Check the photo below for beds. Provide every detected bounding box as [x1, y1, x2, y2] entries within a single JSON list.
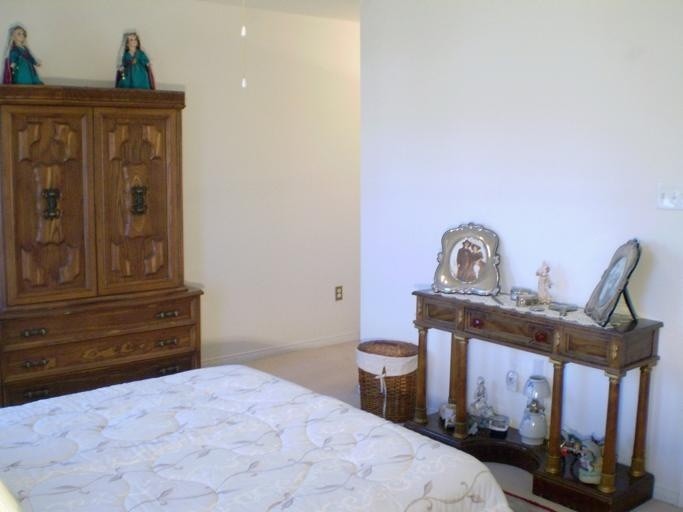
[[0, 364, 513, 511]]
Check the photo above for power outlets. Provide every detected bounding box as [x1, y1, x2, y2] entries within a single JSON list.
[[335, 285, 343, 300]]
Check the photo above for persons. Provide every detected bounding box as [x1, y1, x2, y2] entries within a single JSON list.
[[456, 240, 484, 282], [114, 32, 155, 89], [4, 23, 44, 85], [536, 261, 553, 304]]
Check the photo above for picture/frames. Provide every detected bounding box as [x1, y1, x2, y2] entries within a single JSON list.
[[583, 238, 640, 326], [431, 222, 502, 296]]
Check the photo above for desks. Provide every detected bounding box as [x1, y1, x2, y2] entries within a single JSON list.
[[410, 287, 665, 493]]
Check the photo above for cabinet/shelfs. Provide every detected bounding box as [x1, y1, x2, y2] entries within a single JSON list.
[[1, 82, 201, 412]]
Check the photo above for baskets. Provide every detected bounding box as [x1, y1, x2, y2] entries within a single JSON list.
[[354, 341, 418, 423]]
